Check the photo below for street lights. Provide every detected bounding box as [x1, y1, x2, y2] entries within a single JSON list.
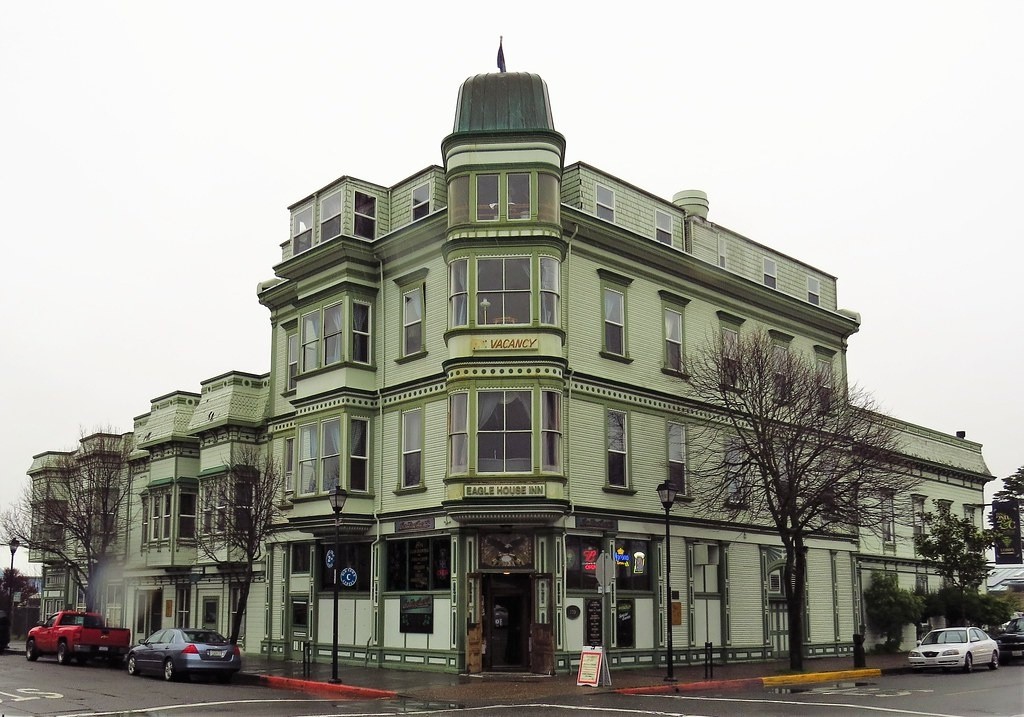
[[327, 485, 348, 685], [656, 479, 681, 683], [7, 538, 21, 620]]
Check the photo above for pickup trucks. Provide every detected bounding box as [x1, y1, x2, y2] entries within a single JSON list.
[[26, 610, 131, 665]]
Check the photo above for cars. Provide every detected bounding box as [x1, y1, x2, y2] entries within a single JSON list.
[[996, 615, 1024, 663], [908, 627, 999, 672], [126, 627, 241, 681]]
